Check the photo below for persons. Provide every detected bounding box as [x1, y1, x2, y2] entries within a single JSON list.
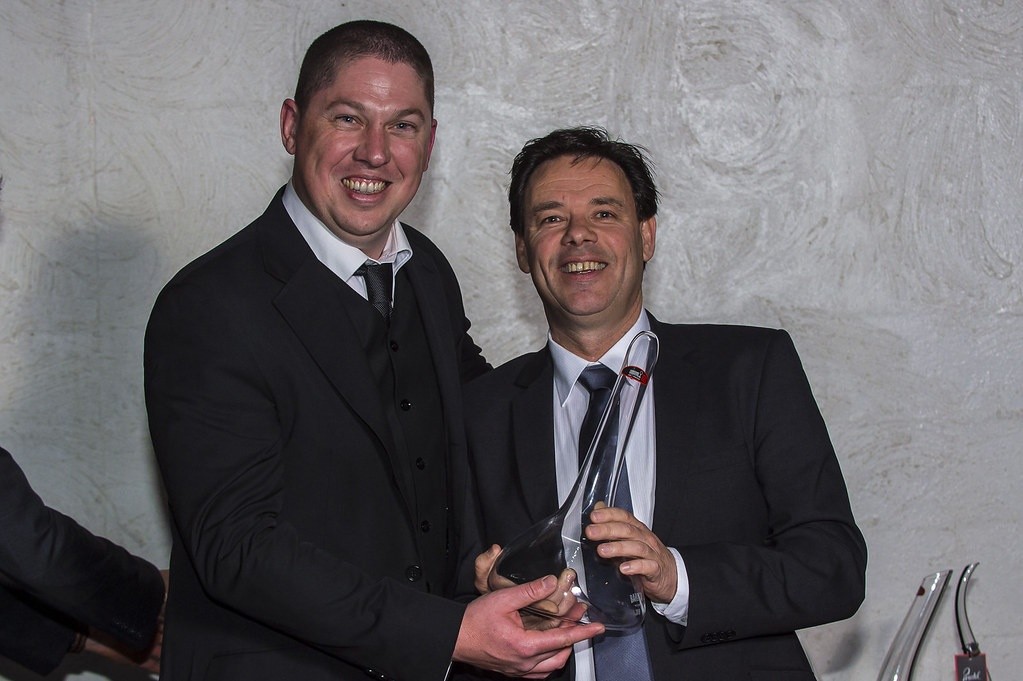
[[0, 447, 165, 676], [462, 126, 867, 681], [144, 20, 606, 681]]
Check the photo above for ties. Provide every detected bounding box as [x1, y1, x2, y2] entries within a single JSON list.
[[576, 364, 653, 681], [352, 263, 393, 324]]
